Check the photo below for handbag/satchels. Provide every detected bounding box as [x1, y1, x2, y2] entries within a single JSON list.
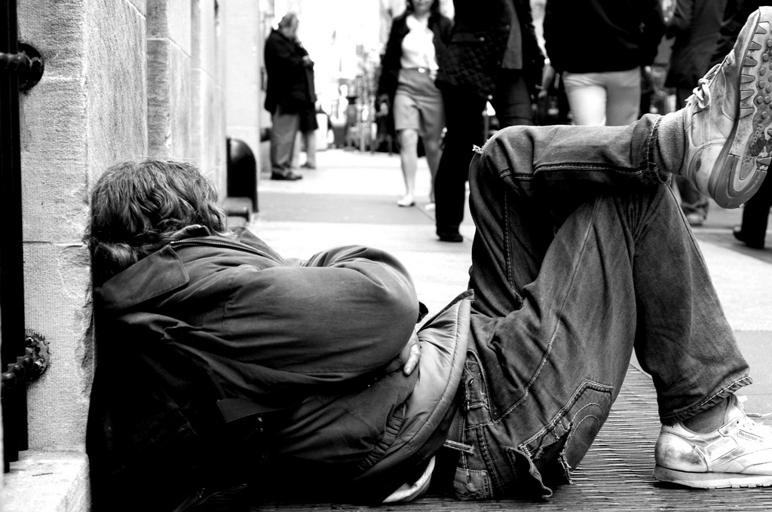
[[89, 317, 277, 511]]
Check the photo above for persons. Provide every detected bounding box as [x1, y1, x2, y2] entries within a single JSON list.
[[541, 0, 666, 127], [92, 4, 772, 507], [263, 12, 319, 183], [373, 1, 453, 208], [732, 158, 771, 251], [434, 0, 544, 243], [661, 0, 772, 227]]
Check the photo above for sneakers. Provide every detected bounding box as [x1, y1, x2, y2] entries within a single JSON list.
[[399, 196, 414, 207], [654, 394, 772, 489], [684, 7, 771, 209], [733, 226, 764, 249], [437, 230, 463, 242], [273, 169, 300, 180]]
